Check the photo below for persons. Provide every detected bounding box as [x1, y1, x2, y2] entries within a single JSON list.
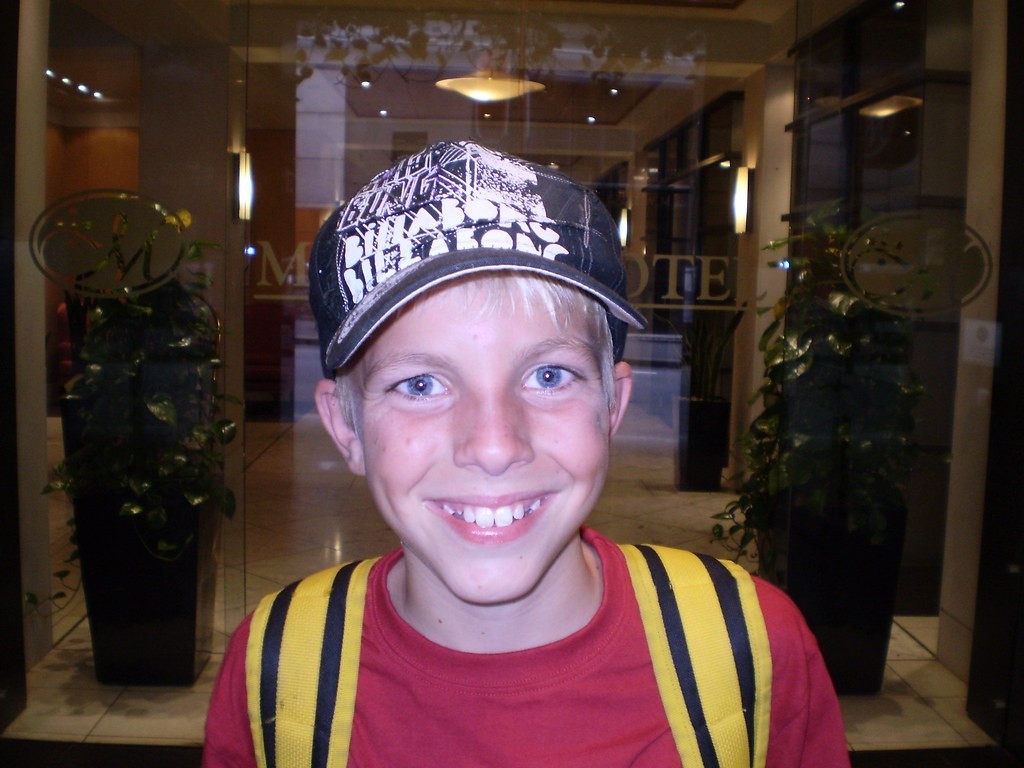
[[199, 142, 850, 768]]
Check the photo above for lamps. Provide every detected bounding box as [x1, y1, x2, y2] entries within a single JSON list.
[[234, 151, 251, 221], [618, 208, 630, 246], [732, 167, 755, 235], [434, 45, 545, 102]]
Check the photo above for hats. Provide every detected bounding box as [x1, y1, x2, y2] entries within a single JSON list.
[[309, 139, 650, 383]]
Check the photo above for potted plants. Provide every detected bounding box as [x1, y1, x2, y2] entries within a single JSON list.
[[24, 229, 240, 685], [708, 196, 928, 695]]
